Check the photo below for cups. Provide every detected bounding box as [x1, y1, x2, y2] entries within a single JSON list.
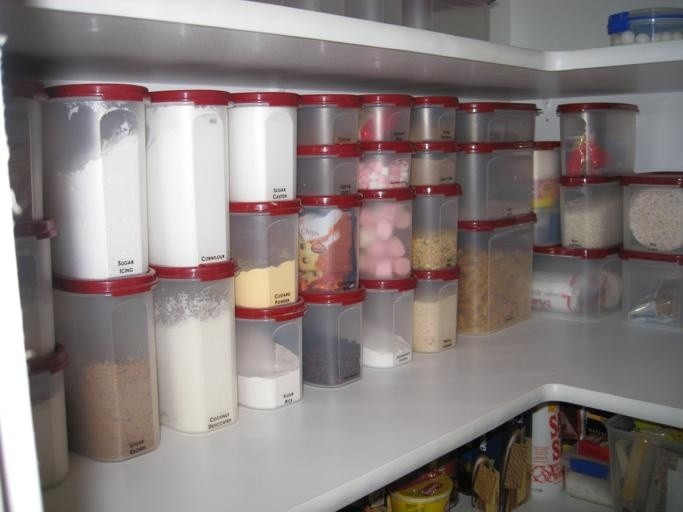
[[387, 471, 455, 512]]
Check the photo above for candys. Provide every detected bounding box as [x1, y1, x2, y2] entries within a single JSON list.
[[357, 158, 411, 190], [359, 203, 413, 277]]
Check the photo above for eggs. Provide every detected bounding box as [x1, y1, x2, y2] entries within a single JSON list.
[[611, 30, 683, 46]]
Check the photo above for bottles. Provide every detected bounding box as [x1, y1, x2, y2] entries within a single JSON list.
[[362, 192, 414, 278], [53, 276, 160, 465], [361, 278, 417, 370], [415, 94, 460, 146], [414, 269, 461, 354], [298, 90, 360, 145], [528, 401, 565, 497], [13, 226, 59, 358], [43, 83, 148, 276], [412, 141, 462, 189], [3, 79, 43, 222], [152, 261, 239, 433], [229, 205, 302, 307], [298, 286, 366, 389], [297, 142, 361, 196], [150, 88, 232, 267], [294, 192, 362, 293], [414, 187, 460, 271], [236, 306, 308, 413], [356, 92, 413, 143], [232, 91, 301, 202], [27, 353, 71, 492], [359, 143, 413, 191]]
